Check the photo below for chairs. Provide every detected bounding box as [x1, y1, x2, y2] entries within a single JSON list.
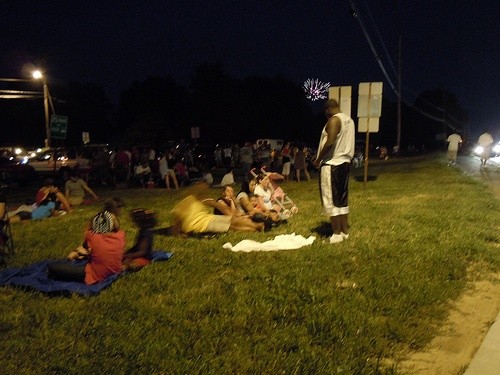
[[0, 206, 15, 265]]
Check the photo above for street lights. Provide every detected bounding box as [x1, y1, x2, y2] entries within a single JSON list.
[[28, 68, 52, 148]]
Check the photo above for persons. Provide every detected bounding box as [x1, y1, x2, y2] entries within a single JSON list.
[[314, 99, 355, 243], [0, 139, 401, 287], [446, 130, 462, 167], [479, 129, 494, 167]]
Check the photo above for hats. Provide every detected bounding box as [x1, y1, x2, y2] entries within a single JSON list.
[[92, 210, 114, 234]]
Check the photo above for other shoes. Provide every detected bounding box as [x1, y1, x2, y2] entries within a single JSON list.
[[340, 231, 350, 239], [322, 234, 343, 245]]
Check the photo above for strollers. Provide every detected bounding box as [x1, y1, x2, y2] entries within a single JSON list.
[[250, 165, 298, 218]]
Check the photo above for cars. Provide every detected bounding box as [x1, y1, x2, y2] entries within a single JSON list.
[[26, 146, 78, 173], [475, 142, 500, 158]]
